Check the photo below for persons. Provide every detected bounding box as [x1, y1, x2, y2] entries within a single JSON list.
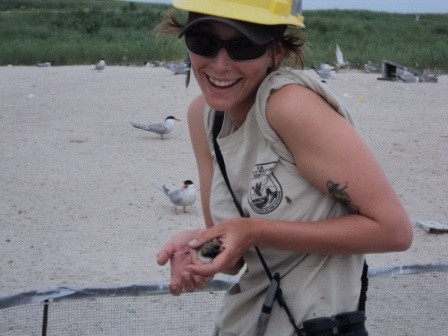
[[148, 2, 415, 336]]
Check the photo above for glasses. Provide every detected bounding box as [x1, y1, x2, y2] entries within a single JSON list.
[[184, 28, 272, 60]]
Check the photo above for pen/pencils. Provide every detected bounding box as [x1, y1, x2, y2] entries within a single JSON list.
[[254, 271, 281, 336]]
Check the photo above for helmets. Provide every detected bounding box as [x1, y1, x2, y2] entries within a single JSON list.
[[170, 0, 306, 27]]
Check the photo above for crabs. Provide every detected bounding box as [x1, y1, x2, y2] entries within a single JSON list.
[[196, 241, 226, 265]]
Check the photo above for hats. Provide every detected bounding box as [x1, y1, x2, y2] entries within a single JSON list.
[[179, 11, 288, 45]]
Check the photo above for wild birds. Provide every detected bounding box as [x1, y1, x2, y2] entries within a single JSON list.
[[130, 115, 184, 140], [90, 58, 106, 71], [312, 60, 349, 84], [163, 180, 197, 215]]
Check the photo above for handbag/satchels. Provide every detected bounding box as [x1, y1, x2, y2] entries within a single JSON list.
[[301, 311, 369, 336]]
